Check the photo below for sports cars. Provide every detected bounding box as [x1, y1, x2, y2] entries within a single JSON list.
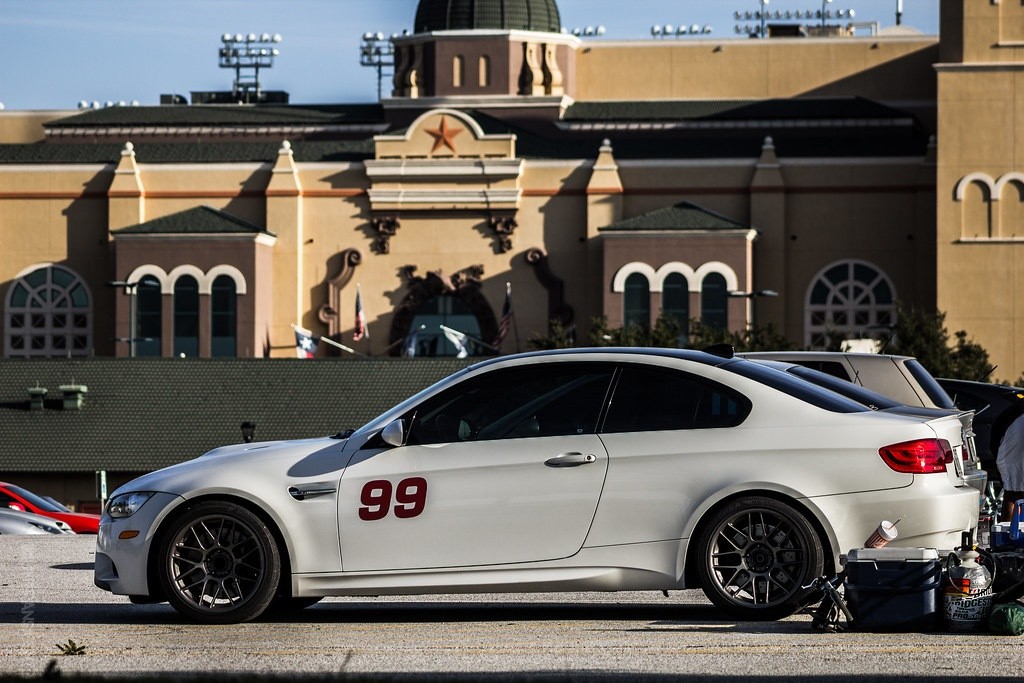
[[92, 344, 978, 624]]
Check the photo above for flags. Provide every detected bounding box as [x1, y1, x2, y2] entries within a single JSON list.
[[493, 295, 512, 350], [294, 330, 320, 358], [352, 293, 365, 343], [444, 328, 468, 358], [404, 333, 417, 358]]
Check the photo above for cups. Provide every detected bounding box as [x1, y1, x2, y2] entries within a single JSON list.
[[865, 520, 897, 548]]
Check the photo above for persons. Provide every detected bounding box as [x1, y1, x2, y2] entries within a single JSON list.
[[996, 403, 1024, 522]]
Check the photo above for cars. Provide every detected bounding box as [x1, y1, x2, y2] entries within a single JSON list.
[[733, 350, 988, 497], [5, 485, 99, 534], [0, 506, 74, 534], [1, 516, 53, 534]]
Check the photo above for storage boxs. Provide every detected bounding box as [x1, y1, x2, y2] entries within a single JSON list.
[[839, 548, 939, 630]]
